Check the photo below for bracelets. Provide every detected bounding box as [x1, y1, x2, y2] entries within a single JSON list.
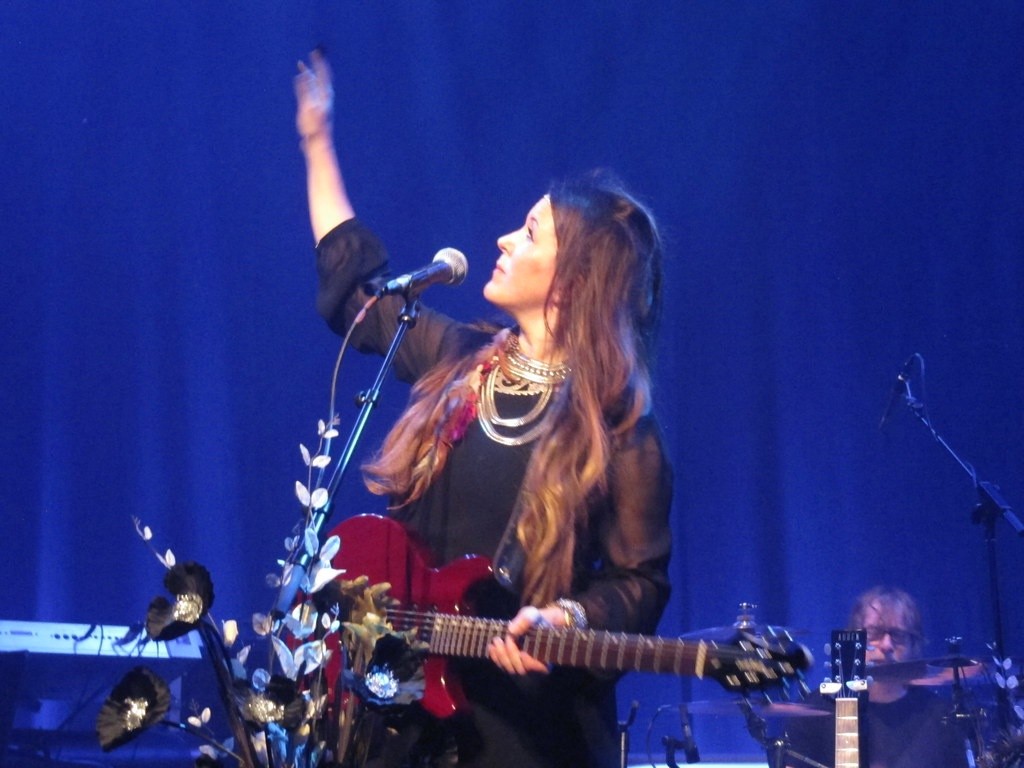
[[549, 598, 588, 632], [297, 132, 321, 148]]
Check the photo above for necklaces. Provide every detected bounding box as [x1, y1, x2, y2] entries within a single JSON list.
[[471, 333, 569, 447]]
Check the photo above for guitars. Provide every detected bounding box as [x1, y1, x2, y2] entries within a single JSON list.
[[820, 627, 874, 768], [284, 514, 813, 720]]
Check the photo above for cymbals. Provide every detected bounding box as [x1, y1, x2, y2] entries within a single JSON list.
[[658, 697, 834, 718], [679, 623, 809, 643], [868, 654, 997, 686]]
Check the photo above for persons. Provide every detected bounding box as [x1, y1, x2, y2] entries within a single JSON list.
[[292, 48, 675, 767], [769, 575, 980, 768]]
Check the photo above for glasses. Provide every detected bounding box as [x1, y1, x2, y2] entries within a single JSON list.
[[865, 625, 916, 645]]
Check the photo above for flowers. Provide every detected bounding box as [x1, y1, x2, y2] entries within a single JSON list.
[[96, 411, 427, 768]]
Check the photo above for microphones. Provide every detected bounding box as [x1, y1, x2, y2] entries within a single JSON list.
[[878, 357, 914, 431], [678, 704, 698, 763], [378, 247, 469, 296]]
